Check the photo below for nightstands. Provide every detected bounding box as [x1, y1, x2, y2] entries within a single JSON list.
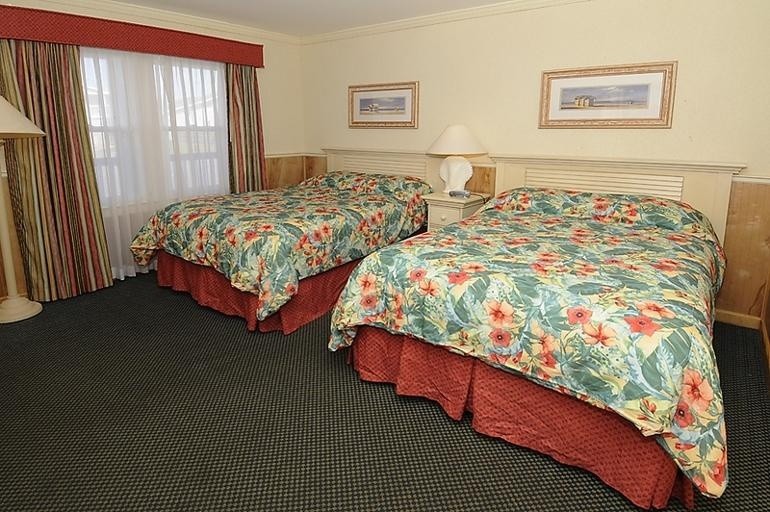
[[420, 190, 491, 232]]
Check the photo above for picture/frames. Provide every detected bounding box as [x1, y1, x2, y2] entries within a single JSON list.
[[348, 81, 419, 130], [536, 62, 679, 129]]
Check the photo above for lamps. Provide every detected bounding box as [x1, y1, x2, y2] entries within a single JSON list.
[[425, 124, 487, 194], [0, 95, 47, 325]]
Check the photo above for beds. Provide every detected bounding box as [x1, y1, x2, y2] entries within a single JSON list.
[[348, 157, 748, 488], [148, 147, 443, 334]]
